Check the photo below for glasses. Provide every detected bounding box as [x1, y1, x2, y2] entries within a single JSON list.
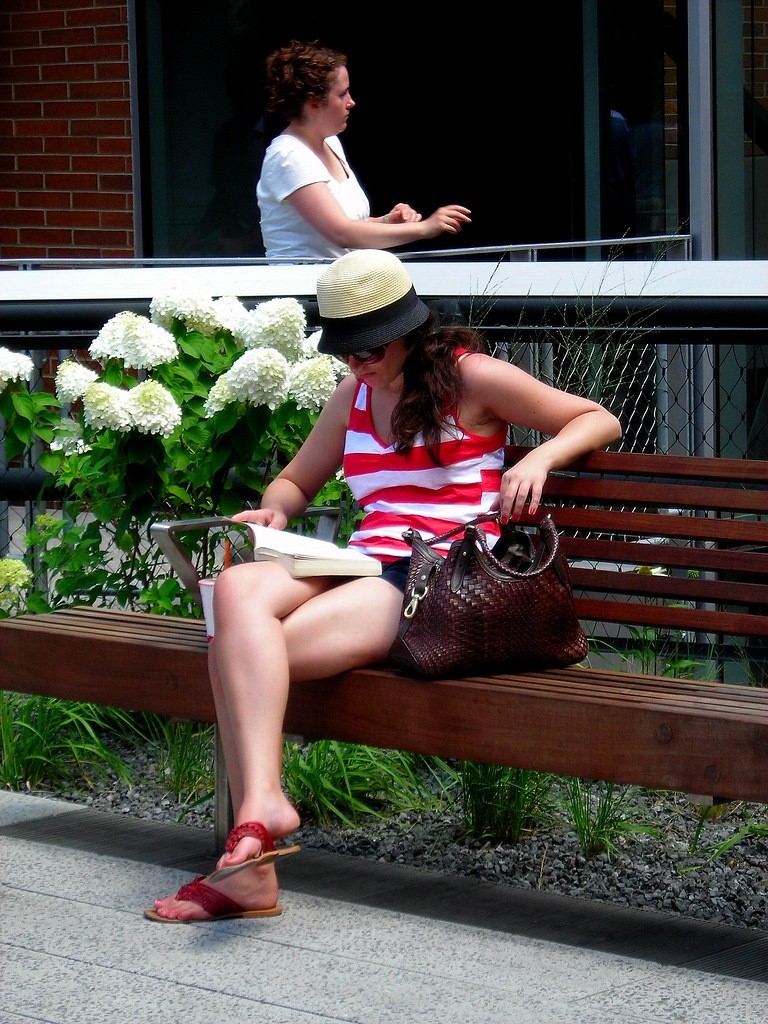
[[332, 342, 397, 364]]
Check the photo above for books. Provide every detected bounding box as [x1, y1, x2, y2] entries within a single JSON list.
[[221, 516, 383, 578]]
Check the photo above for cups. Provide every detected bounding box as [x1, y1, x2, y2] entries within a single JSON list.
[[197, 578, 214, 644]]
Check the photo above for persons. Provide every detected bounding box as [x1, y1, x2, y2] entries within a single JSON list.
[[250, 39, 474, 263], [142, 250, 624, 923]]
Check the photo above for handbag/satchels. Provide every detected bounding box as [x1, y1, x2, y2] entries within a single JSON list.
[[384, 510, 592, 683]]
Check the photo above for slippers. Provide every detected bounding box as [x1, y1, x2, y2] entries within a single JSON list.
[[208, 821, 302, 885], [144, 875, 282, 924]]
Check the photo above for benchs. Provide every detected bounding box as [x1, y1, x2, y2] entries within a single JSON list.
[[0, 442, 768, 858]]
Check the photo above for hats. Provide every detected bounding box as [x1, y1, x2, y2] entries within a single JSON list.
[[318, 247, 430, 352]]
